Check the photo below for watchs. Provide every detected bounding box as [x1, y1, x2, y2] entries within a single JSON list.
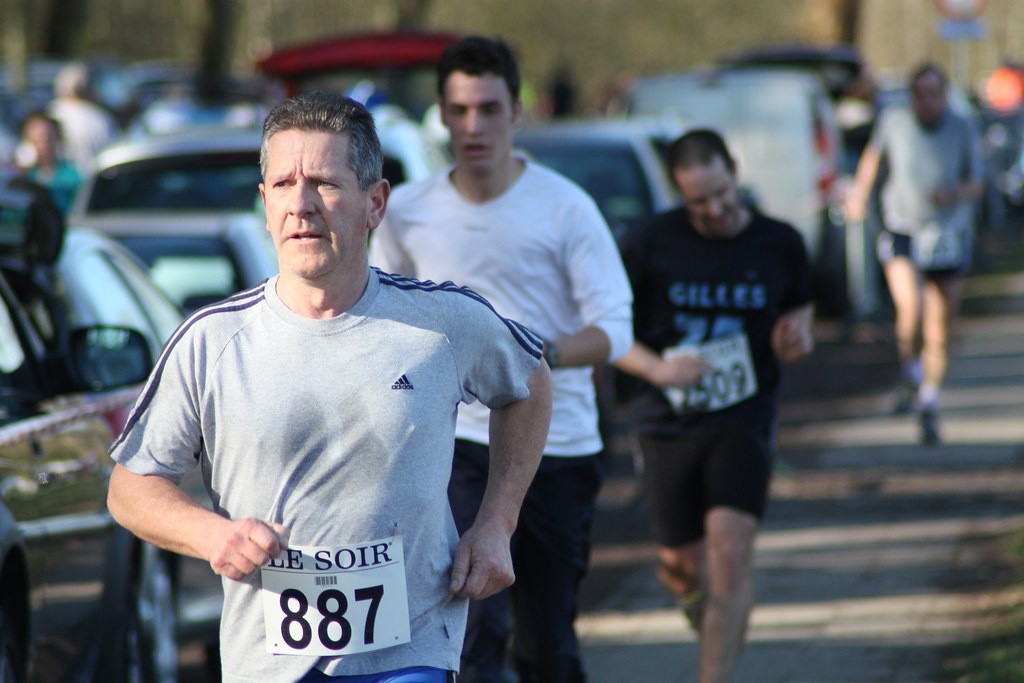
[[544, 341, 560, 369]]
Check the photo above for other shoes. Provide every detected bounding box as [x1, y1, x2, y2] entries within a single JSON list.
[[915, 398, 940, 446], [894, 360, 921, 414], [683, 582, 707, 635]]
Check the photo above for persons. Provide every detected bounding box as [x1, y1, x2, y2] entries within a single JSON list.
[[612, 130, 816, 683], [106, 90, 553, 683], [834, 64, 878, 152], [22, 111, 81, 216], [850, 67, 985, 445], [46, 62, 118, 166], [370, 35, 634, 683]]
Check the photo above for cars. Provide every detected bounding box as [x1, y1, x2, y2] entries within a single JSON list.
[[1, 29, 1024, 683]]
